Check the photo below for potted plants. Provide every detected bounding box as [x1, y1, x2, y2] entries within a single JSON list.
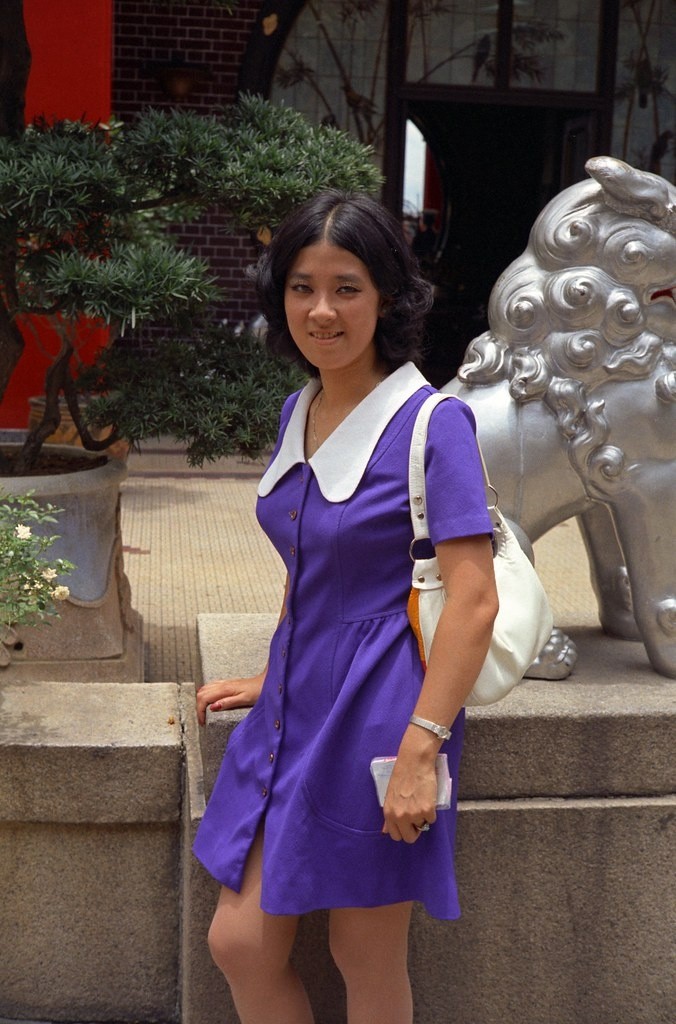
[[1, 91, 390, 603]]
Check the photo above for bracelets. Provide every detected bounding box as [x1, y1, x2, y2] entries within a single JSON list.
[[408, 715, 451, 740]]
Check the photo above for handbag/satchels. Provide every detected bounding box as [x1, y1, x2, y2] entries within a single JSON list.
[[407, 392, 553, 709]]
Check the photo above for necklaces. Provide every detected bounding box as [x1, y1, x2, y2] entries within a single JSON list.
[[311, 374, 386, 451]]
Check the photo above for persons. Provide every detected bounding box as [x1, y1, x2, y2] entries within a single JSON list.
[[195, 186, 500, 1023]]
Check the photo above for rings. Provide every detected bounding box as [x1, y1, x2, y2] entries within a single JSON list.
[[417, 823, 430, 832]]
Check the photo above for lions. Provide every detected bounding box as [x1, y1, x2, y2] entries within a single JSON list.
[[440, 157, 676, 680]]
